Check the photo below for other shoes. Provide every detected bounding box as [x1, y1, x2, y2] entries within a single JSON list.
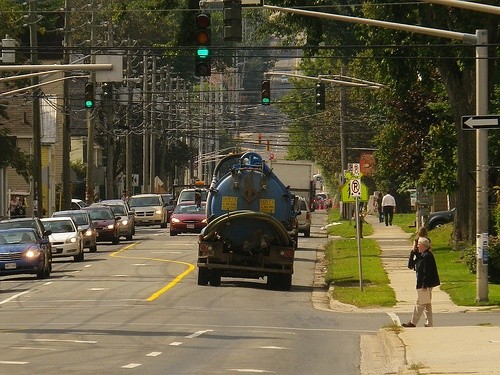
[[402, 321, 416, 327], [424, 323, 428, 327]]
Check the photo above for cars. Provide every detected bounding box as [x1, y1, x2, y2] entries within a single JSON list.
[[427, 207, 455, 231], [296, 198, 312, 238], [70, 188, 208, 245], [168, 201, 208, 237], [51, 210, 98, 252], [0, 214, 53, 279], [38, 217, 87, 262]]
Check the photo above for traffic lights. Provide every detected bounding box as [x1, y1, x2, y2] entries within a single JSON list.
[[102, 82, 114, 101], [83, 81, 95, 111], [194, 12, 212, 77], [261, 79, 271, 105]]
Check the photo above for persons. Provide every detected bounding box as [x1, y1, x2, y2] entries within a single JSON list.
[[382, 190, 395, 226], [8, 194, 19, 219], [413, 227, 432, 253], [359, 202, 364, 213], [377, 192, 384, 223], [401, 237, 440, 327], [19, 195, 28, 218]]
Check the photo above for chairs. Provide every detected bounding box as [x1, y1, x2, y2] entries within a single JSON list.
[[63, 225, 73, 230], [181, 208, 187, 213], [0, 235, 7, 245], [77, 218, 83, 223], [22, 233, 31, 241], [115, 208, 120, 213], [102, 211, 109, 217]]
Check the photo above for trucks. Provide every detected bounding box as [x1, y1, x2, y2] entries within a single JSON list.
[[196, 151, 299, 292], [269, 159, 312, 208]]
